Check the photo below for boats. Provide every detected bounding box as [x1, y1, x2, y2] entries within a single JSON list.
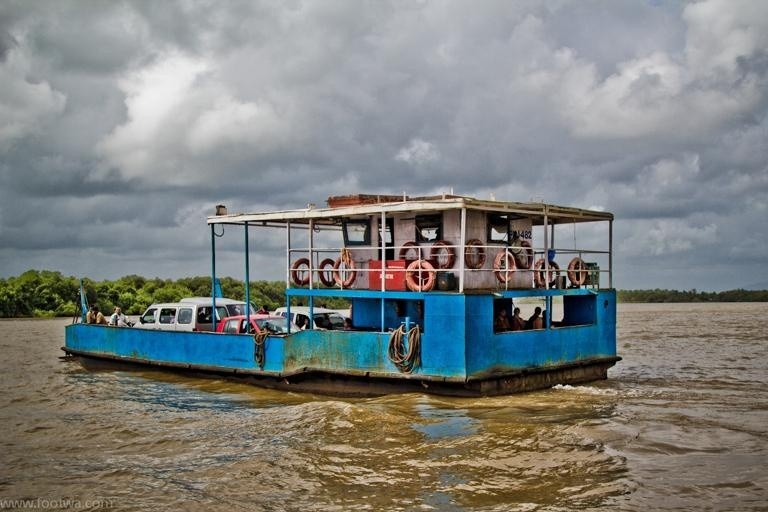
[[60, 194, 621, 400]]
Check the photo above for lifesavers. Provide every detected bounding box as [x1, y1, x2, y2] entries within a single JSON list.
[[429, 240, 455, 269], [405, 260, 437, 292], [333, 257, 356, 287], [399, 242, 423, 269], [319, 258, 338, 287], [534, 259, 560, 286], [291, 258, 314, 285], [515, 241, 533, 269], [465, 239, 486, 271], [567, 258, 586, 286], [494, 251, 517, 283]]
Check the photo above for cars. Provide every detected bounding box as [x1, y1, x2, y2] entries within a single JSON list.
[[131, 296, 348, 337]]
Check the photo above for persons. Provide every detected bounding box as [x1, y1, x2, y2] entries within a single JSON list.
[[91, 306, 109, 326], [296, 302, 304, 306], [110, 306, 131, 327], [85, 304, 95, 325], [319, 303, 327, 308], [237, 311, 351, 337], [511, 307, 524, 330], [363, 222, 383, 242], [415, 225, 427, 241], [143, 306, 217, 324], [541, 310, 554, 330], [526, 306, 543, 330], [493, 308, 512, 332], [254, 305, 269, 314]]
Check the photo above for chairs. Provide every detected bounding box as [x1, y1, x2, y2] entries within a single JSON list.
[[297, 317, 333, 330], [226, 323, 283, 333], [161, 312, 217, 323]]
